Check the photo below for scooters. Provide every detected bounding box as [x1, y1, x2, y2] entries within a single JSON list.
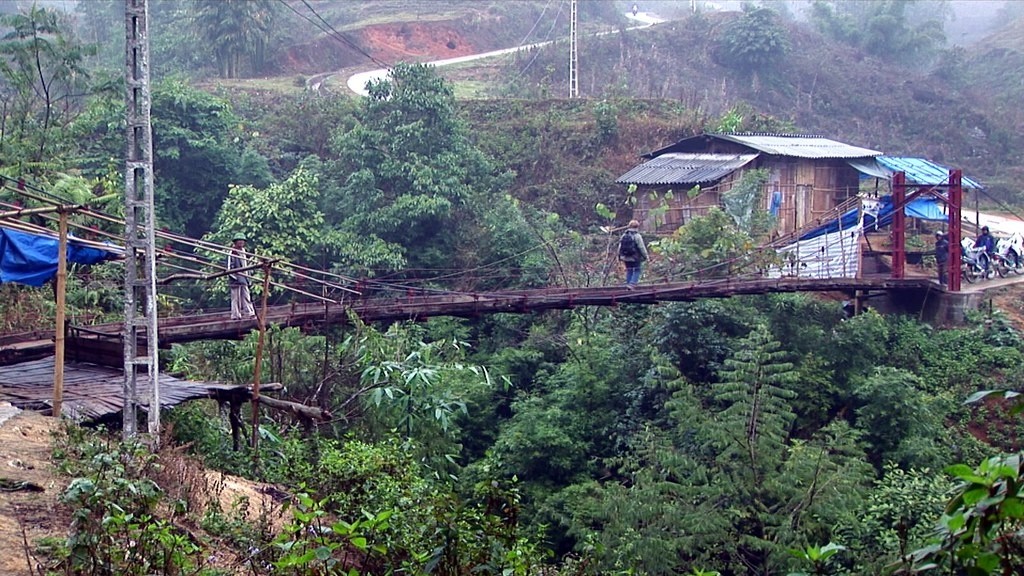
[[961, 239, 1024, 285]]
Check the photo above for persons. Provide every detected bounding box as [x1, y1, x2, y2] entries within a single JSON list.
[[973, 225, 995, 279], [616, 220, 650, 292], [226, 232, 258, 321], [935, 230, 949, 284]]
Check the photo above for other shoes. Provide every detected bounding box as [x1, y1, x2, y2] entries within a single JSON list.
[[626, 285, 635, 291], [250, 315, 257, 319]]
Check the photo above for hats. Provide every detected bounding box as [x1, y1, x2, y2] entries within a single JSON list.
[[232, 233, 248, 242]]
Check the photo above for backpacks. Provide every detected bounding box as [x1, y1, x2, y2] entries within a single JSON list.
[[620, 230, 637, 257]]
